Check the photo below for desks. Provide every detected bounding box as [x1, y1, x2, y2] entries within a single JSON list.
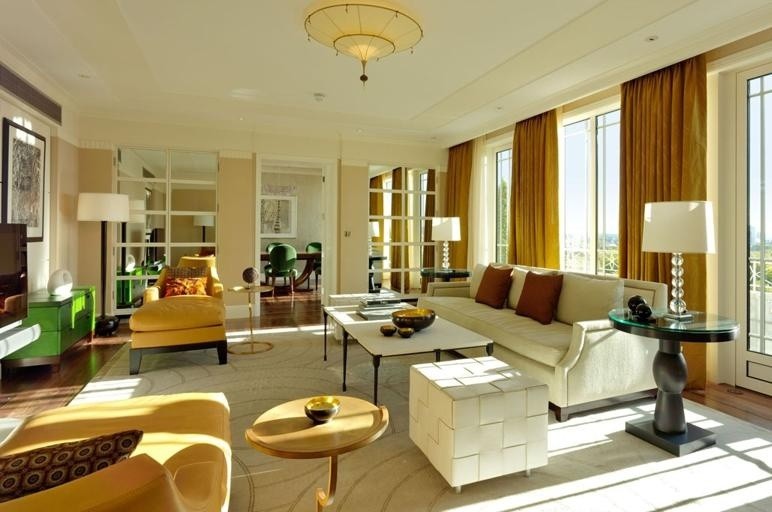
[[421, 268, 472, 282], [607, 309, 739, 455], [244, 395, 389, 511], [228, 285, 275, 354], [178, 257, 217, 268], [260, 253, 387, 292], [323, 302, 494, 406]]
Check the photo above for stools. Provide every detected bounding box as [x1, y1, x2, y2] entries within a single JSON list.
[[409, 357, 549, 494]]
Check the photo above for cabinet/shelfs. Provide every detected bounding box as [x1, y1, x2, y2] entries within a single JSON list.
[[0, 286, 96, 373]]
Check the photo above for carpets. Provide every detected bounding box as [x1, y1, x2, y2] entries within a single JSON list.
[[65, 323, 771, 512]]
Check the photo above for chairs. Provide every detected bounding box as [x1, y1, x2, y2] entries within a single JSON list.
[[264, 244, 297, 294], [306, 243, 321, 288], [314, 268, 321, 290], [0, 391, 230, 509], [263, 242, 287, 286], [129, 266, 228, 373]]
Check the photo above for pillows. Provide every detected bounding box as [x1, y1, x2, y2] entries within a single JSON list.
[[165, 267, 208, 278], [475, 264, 513, 309], [0, 431, 143, 503], [515, 271, 564, 325], [163, 277, 208, 296], [470, 264, 514, 299], [555, 273, 624, 324], [508, 266, 558, 310]]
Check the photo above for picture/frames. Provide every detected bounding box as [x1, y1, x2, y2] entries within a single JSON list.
[[260, 195, 298, 238], [2, 117, 45, 242]]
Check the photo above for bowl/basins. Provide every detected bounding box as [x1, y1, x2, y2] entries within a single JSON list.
[[380, 325, 397, 337], [398, 327, 415, 338], [391, 308, 436, 332], [303, 396, 341, 424]]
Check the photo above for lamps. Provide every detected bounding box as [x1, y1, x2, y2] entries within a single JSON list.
[[369, 221, 380, 256], [192, 216, 214, 242], [431, 216, 462, 272], [303, 1, 425, 86], [641, 200, 717, 320], [77, 192, 129, 336]]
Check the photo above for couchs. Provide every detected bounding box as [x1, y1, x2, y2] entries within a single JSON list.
[[418, 263, 670, 421]]
